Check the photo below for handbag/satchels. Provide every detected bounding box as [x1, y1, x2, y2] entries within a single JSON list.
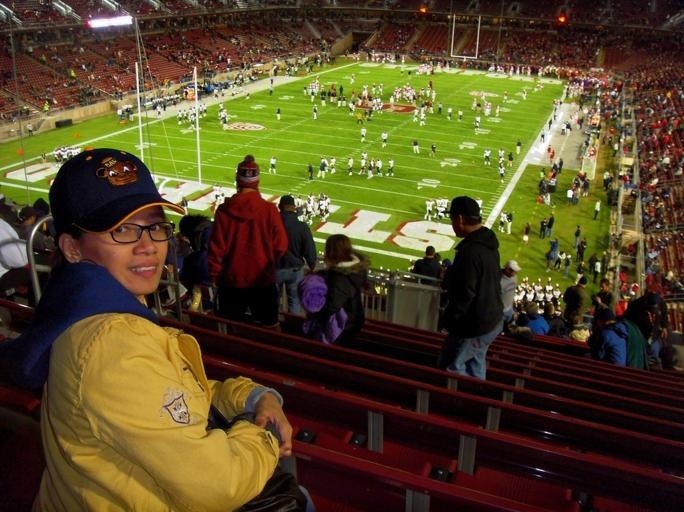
[[238, 466, 307, 512]]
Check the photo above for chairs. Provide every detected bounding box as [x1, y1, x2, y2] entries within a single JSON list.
[[2, 3, 337, 125], [2, 198, 681, 511], [632, 83, 683, 346], [333, 1, 683, 92]]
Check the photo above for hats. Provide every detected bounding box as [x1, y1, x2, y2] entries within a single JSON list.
[[441, 196, 480, 216], [279, 195, 294, 209], [505, 260, 523, 272], [236, 155, 259, 189], [49, 149, 185, 234]]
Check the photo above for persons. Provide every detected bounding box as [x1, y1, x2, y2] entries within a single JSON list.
[[143, 154, 505, 380], [0, 1, 684, 181], [1, 145, 293, 512], [497, 178, 683, 369], [1, 195, 57, 325]]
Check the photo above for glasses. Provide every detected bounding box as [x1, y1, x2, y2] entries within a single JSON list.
[[110, 222, 174, 243]]
